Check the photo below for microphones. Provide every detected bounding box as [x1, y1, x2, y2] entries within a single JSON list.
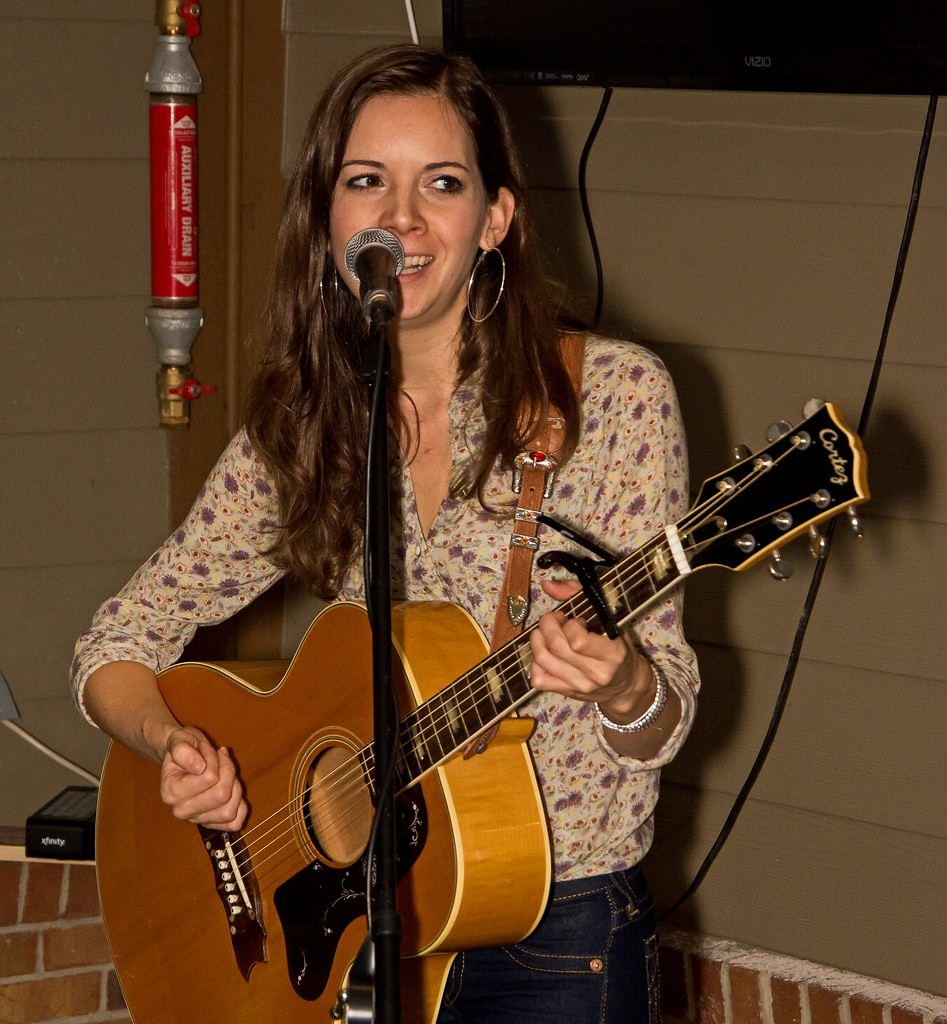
[[344, 228, 405, 320]]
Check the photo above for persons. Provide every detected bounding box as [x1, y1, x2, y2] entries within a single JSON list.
[[68, 41, 702, 1024]]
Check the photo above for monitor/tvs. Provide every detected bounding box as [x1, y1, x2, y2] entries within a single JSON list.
[[443, 0, 947, 96]]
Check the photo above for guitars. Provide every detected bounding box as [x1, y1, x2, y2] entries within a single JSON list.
[[94, 399, 870, 1024]]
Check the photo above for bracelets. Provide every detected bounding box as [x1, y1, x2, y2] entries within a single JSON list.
[[594, 662, 669, 734]]
